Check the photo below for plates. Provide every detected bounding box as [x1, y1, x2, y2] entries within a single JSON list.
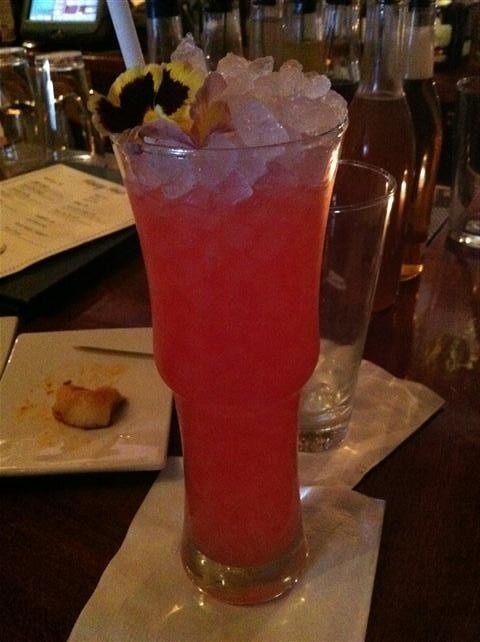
[[0, 323, 177, 472]]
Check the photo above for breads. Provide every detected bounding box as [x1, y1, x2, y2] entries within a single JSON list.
[[52, 379, 128, 428]]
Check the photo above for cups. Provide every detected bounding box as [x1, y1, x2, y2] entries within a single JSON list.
[[35, 48, 105, 162], [0, 45, 48, 174], [445, 76, 480, 250], [113, 102, 350, 607], [301, 148, 398, 458]]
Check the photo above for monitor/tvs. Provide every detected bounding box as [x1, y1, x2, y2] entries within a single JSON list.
[[21, 0, 104, 30]]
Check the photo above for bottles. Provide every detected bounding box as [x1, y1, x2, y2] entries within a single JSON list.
[[147, 0, 443, 311]]
